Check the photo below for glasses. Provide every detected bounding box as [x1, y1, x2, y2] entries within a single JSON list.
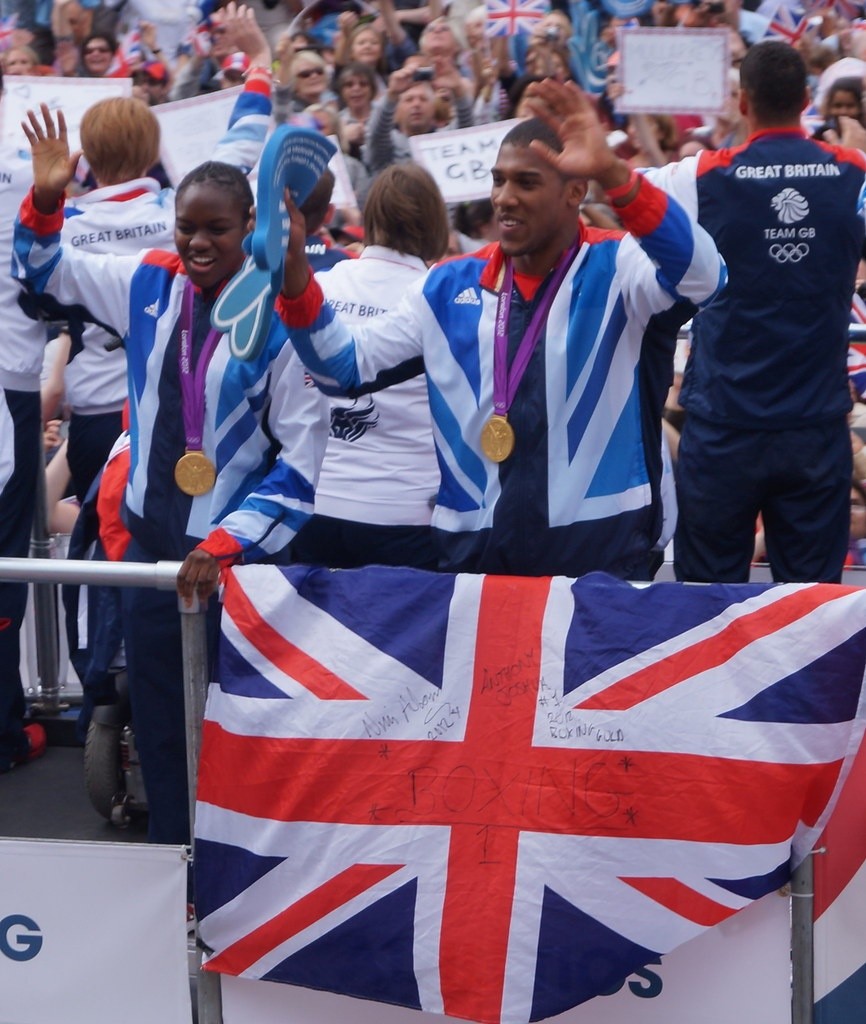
[[296, 67, 325, 78], [850, 504, 866, 515], [84, 46, 112, 53]]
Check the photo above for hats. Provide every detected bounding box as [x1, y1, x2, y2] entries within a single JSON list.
[[221, 52, 250, 73], [131, 61, 169, 82]]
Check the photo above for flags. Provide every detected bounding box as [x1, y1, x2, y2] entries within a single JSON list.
[[197, 558, 866, 1024]]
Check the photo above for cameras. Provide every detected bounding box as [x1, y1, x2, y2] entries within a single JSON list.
[[414, 67, 435, 82]]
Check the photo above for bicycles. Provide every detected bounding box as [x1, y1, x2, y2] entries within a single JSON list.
[[82, 663, 154, 834]]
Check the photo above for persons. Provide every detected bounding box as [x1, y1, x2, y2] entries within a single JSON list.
[[2, 0, 866, 811]]
